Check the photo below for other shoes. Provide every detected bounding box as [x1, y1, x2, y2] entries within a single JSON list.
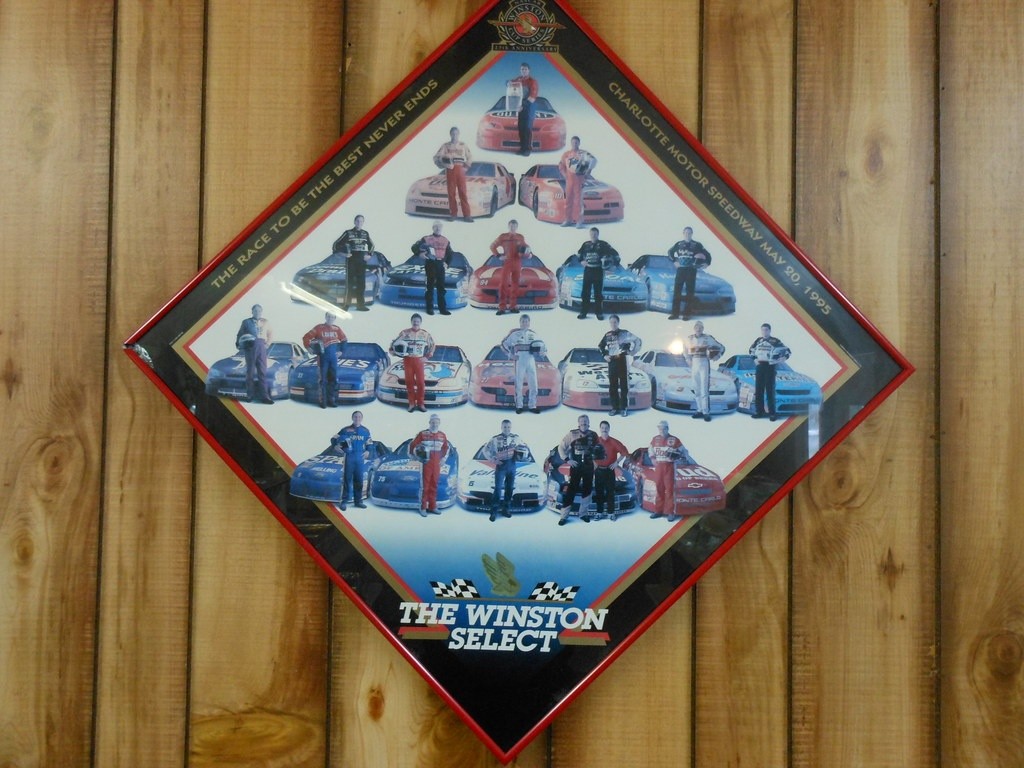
[[340, 501, 676, 527], [517, 149, 531, 157], [342, 303, 691, 321], [246, 394, 777, 425], [560, 220, 583, 228], [447, 215, 475, 223]]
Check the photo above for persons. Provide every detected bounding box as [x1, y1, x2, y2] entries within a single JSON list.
[[500, 314, 547, 414], [433, 127, 474, 222], [749, 324, 792, 422], [682, 321, 725, 421], [331, 411, 373, 511], [389, 313, 435, 413], [483, 419, 532, 521], [411, 221, 454, 315], [490, 220, 533, 315], [558, 414, 607, 525], [408, 415, 448, 516], [235, 304, 275, 405], [594, 421, 628, 521], [667, 227, 712, 321], [649, 421, 683, 522], [302, 310, 347, 408], [598, 315, 642, 417], [506, 63, 538, 156], [577, 227, 621, 320], [559, 136, 597, 229], [333, 215, 375, 311]]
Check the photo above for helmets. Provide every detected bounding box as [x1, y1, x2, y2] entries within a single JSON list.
[[592, 443, 607, 460], [310, 339, 325, 355], [439, 154, 455, 170], [529, 340, 545, 356], [337, 242, 353, 258], [692, 252, 707, 270], [419, 243, 437, 261], [337, 436, 353, 454], [600, 254, 615, 270], [769, 346, 788, 365], [392, 340, 410, 358], [705, 345, 721, 360], [515, 445, 530, 463], [414, 445, 430, 464], [238, 333, 258, 350], [618, 341, 635, 355], [519, 244, 531, 259]]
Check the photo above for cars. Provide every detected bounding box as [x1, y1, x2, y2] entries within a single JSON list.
[[288, 343, 391, 403], [379, 252, 473, 310], [469, 251, 558, 309], [289, 441, 392, 502], [543, 445, 636, 516], [634, 348, 738, 415], [376, 345, 472, 407], [477, 96, 566, 152], [457, 442, 548, 513], [518, 163, 624, 224], [623, 447, 726, 515], [555, 253, 648, 311], [626, 254, 736, 312], [404, 161, 516, 218], [558, 348, 652, 410], [370, 438, 459, 508], [469, 344, 563, 407], [717, 354, 823, 416], [205, 342, 309, 399], [289, 250, 392, 306]]
